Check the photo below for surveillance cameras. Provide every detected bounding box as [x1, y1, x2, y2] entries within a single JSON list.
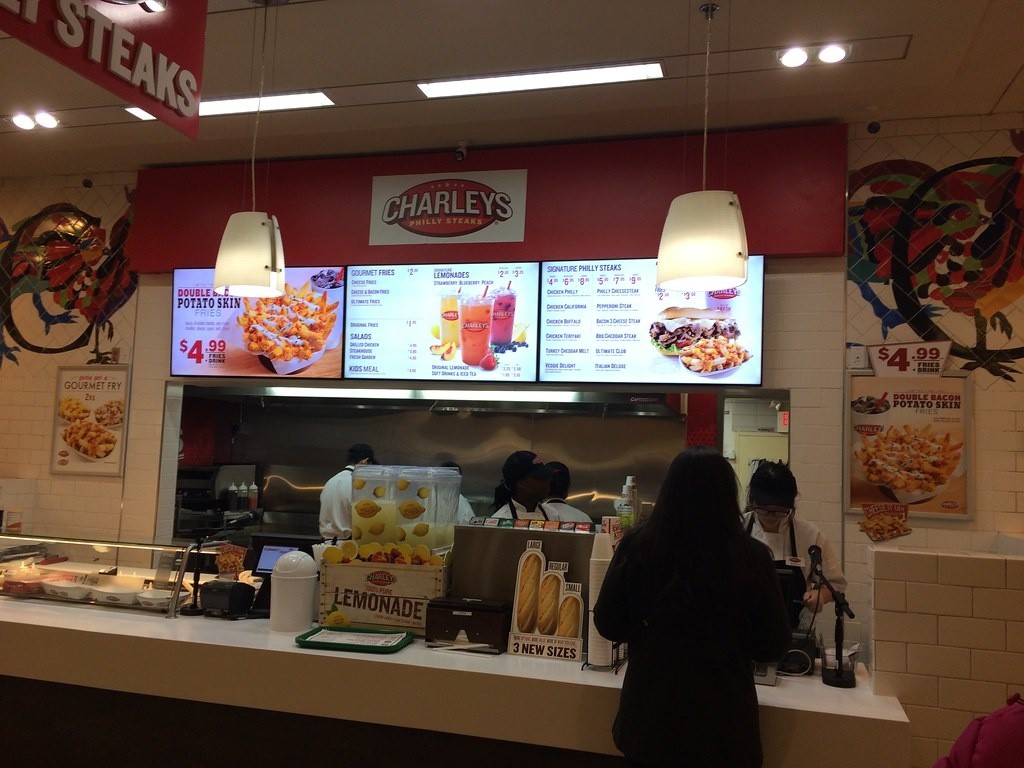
[[453, 147, 466, 160]]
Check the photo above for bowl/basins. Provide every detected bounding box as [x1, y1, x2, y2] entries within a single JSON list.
[[680, 346, 753, 379], [136, 589, 191, 613], [256, 347, 324, 375], [90, 586, 141, 605], [56, 412, 124, 462], [41, 580, 91, 600]]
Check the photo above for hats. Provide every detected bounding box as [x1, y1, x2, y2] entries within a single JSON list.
[[502, 449, 552, 481], [351, 443, 381, 466]]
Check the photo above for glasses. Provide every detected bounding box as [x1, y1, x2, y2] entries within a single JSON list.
[[753, 503, 791, 518]]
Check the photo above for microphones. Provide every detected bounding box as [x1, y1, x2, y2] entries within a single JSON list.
[[808, 544, 823, 574], [228, 511, 259, 526]]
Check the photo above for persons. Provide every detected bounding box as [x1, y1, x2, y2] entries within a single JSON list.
[[489, 451, 561, 521], [592, 447, 793, 767], [739, 461, 848, 635], [319, 444, 374, 543], [440, 462, 476, 523], [545, 461, 594, 523], [932, 693, 1024, 768]]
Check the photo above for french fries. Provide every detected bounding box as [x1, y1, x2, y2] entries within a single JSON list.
[[681, 336, 750, 372], [238, 282, 339, 360], [855, 424, 963, 492], [58, 396, 116, 457]]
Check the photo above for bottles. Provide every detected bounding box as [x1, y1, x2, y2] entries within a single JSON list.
[[616, 476, 642, 529], [227, 482, 238, 510], [238, 482, 248, 511], [249, 482, 258, 510]]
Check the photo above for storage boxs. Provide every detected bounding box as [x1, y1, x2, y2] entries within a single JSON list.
[[319, 562, 445, 637]]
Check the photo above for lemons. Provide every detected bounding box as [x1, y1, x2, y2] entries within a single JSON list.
[[325, 611, 351, 626], [322, 478, 444, 565]]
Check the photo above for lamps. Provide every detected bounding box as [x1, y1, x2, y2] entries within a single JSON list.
[[657, 4, 749, 293], [214, 0, 287, 299]]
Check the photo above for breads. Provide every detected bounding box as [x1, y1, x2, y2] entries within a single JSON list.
[[517, 554, 580, 638]]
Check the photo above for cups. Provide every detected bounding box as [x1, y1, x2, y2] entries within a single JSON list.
[[588, 516, 628, 666], [440, 290, 517, 366], [310, 274, 345, 349], [851, 400, 891, 446]]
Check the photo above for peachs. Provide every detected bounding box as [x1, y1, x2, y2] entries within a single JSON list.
[[429, 342, 456, 360]]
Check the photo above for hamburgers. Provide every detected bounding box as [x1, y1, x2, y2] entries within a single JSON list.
[[649, 306, 741, 355]]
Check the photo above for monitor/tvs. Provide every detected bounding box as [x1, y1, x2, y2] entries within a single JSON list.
[[251, 545, 301, 577]]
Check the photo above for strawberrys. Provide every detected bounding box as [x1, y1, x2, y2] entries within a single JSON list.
[[480, 355, 497, 370]]
